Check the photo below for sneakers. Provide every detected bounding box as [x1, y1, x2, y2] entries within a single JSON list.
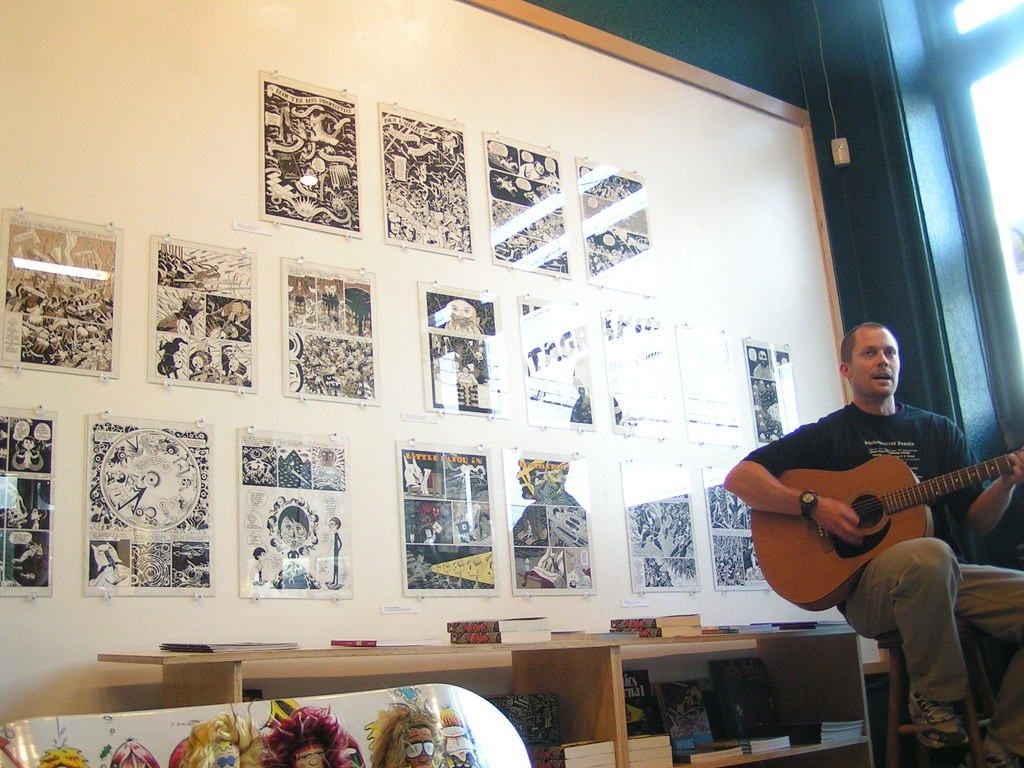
[[973, 736, 1021, 768], [907, 688, 969, 749]]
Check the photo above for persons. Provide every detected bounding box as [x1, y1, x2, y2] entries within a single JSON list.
[[722, 319, 1023, 767], [512, 458, 588, 554]]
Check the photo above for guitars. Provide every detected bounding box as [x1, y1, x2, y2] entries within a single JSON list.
[[751, 448, 1024, 618]]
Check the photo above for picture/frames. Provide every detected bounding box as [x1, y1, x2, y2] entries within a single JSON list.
[[0, 69, 798, 599]]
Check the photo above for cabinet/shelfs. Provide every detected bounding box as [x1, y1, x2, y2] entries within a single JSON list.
[[97, 622, 877, 768]]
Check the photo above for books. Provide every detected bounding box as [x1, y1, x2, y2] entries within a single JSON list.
[[447, 613, 702, 645], [701, 620, 856, 636], [158, 642, 302, 654], [242, 688, 263, 702], [488, 693, 618, 768], [330, 639, 429, 647], [622, 655, 864, 768]]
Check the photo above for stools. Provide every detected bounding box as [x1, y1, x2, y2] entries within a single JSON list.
[[876, 617, 998, 768]]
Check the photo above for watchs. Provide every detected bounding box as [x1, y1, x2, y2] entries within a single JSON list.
[[799, 490, 819, 521]]
